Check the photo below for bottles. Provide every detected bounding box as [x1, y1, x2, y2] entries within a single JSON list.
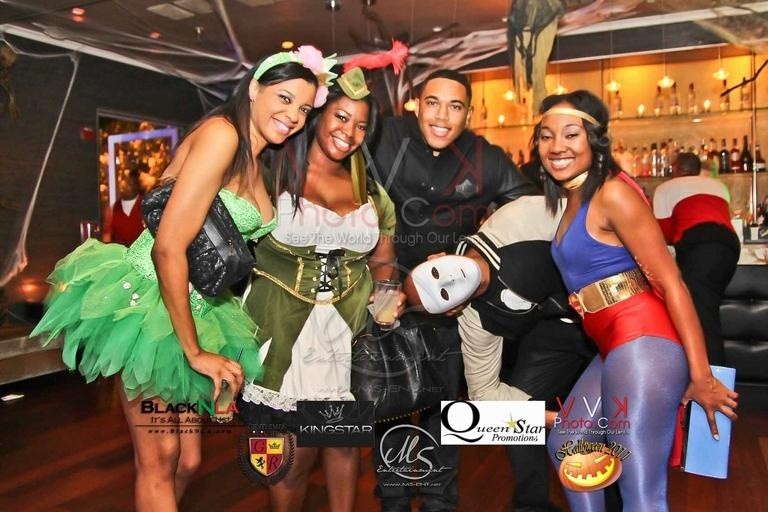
[[654, 86, 665, 116], [505, 134, 767, 175], [741, 78, 750, 108], [720, 79, 731, 111], [80, 215, 97, 242], [687, 83, 697, 113], [614, 90, 622, 120], [479, 96, 489, 129], [670, 82, 681, 114], [520, 96, 530, 130]]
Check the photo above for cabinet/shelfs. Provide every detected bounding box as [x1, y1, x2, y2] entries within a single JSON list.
[[475, 107, 767, 184]]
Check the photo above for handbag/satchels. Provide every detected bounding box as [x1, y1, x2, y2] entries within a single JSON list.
[[141, 181, 253, 297], [348, 313, 463, 427]]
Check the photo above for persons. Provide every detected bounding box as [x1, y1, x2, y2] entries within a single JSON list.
[[527, 90, 739, 512], [339, 70, 545, 512], [32, 50, 319, 512], [652, 151, 741, 368], [235, 70, 407, 511], [404, 195, 627, 512]]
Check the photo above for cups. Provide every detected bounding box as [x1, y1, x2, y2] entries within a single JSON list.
[[374, 280, 400, 326], [216, 378, 234, 423]]
